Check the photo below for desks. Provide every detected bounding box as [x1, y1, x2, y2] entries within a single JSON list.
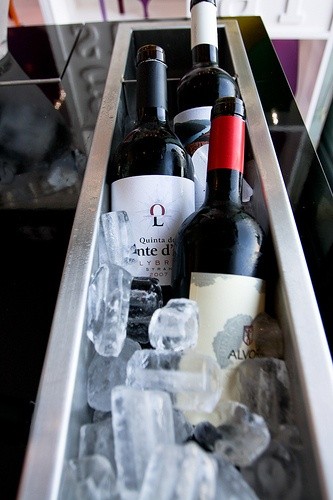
[[0, 13, 332, 500]]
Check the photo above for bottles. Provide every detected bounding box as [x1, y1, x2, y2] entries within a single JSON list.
[[172, 0, 239, 212], [106, 45, 199, 349], [169, 96, 274, 470]]
